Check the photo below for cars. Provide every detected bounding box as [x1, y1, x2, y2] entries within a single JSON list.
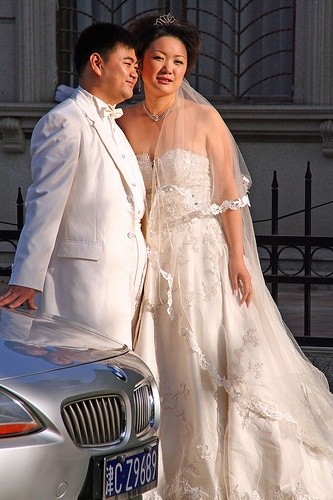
[[0, 305, 161, 500]]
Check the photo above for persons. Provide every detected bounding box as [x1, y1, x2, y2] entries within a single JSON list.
[[114, 12, 333, 500], [0, 22, 146, 355]]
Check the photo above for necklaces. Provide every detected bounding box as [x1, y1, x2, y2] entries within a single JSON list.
[[142, 97, 180, 122]]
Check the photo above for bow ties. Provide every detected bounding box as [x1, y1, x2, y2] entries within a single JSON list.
[[102, 107, 123, 119]]
